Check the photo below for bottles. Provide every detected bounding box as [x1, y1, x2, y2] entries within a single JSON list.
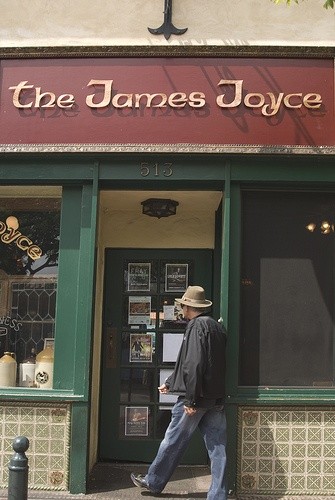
[[0, 351, 17, 388], [19, 348, 39, 388], [34, 337, 54, 389]]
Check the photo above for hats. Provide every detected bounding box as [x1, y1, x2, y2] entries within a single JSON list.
[[174, 285, 213, 308]]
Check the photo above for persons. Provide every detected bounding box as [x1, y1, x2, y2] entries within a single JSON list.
[[138, 321, 147, 335], [131, 286, 230, 499], [131, 338, 145, 360]]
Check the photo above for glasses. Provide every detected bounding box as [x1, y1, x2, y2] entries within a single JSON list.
[[180, 303, 191, 308]]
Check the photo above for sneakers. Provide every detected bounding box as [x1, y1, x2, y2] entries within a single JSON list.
[[130, 472, 161, 494]]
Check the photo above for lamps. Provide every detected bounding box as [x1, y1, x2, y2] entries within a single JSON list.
[[306, 219, 335, 235], [141, 199, 178, 221]]
